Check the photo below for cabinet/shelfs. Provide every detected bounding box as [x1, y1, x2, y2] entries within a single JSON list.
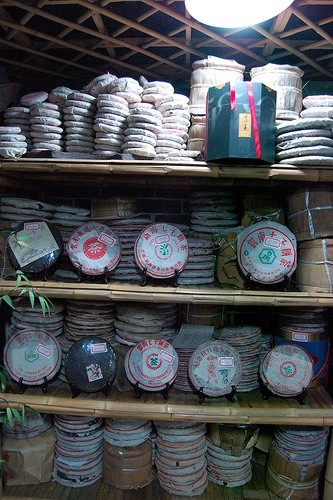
[[0, 158, 333, 500]]
